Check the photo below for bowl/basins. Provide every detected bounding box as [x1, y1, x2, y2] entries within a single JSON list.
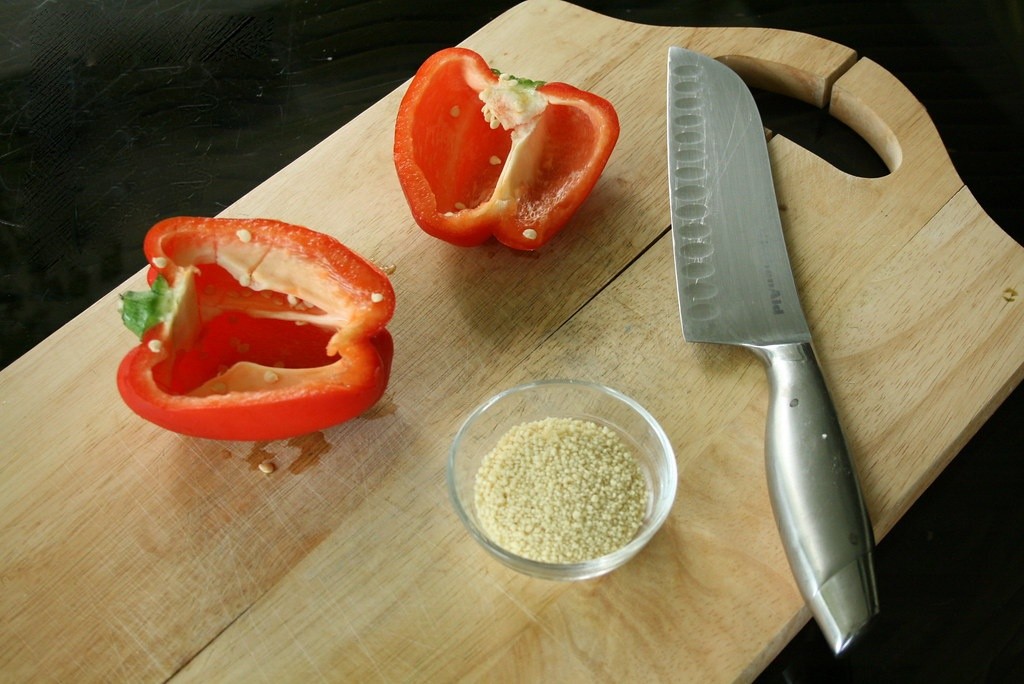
[[444, 377, 680, 581]]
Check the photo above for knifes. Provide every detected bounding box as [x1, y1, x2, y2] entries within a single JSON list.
[[664, 44, 883, 656]]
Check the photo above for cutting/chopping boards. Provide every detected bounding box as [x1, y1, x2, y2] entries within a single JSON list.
[[0, 0, 1024, 684]]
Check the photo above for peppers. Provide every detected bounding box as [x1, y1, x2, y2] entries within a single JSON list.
[[391, 46, 620, 251], [117, 216, 396, 439]]
